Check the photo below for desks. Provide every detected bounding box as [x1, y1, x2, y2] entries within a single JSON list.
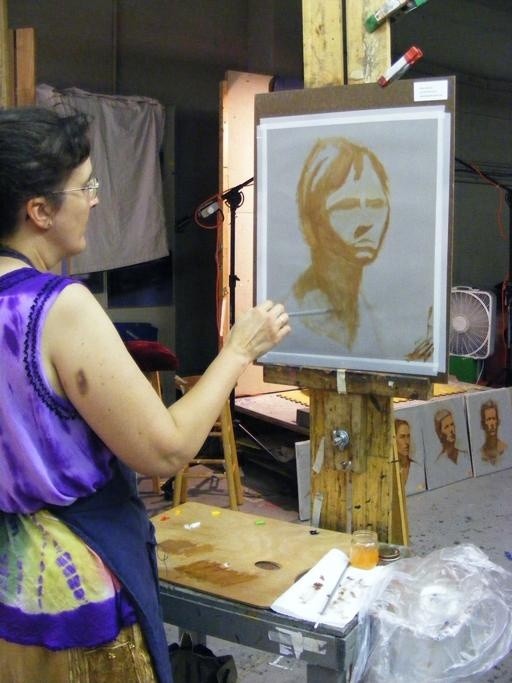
[[145, 502, 413, 683], [233, 381, 489, 479]]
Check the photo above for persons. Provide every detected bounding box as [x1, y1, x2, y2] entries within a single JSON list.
[[479, 400, 508, 464], [293, 137, 392, 344], [0, 101, 292, 683], [393, 419, 424, 496], [427, 409, 472, 487]]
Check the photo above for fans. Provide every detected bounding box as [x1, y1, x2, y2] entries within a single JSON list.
[[448, 285, 496, 359]]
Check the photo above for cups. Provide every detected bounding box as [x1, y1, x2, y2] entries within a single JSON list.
[[350, 529, 379, 570]]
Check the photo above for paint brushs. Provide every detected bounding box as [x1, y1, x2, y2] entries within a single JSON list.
[[288, 308, 340, 318], [314, 559, 351, 627]]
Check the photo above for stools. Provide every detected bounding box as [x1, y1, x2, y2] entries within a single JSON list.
[[117, 339, 179, 494], [171, 377, 245, 510]]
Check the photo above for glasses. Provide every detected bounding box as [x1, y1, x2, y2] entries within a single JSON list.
[[24, 174, 98, 223]]
